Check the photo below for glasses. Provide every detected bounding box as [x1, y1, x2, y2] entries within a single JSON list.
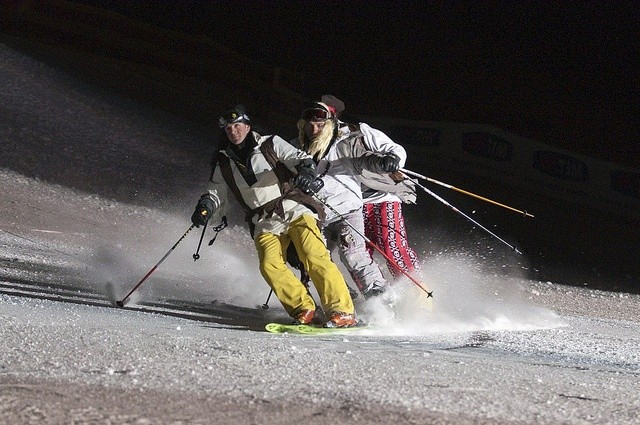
[[218, 112, 250, 128], [302, 109, 328, 121]]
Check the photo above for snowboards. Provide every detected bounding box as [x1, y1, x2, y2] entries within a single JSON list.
[[263, 315, 375, 339]]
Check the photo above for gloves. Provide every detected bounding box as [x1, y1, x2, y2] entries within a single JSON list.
[[380, 153, 401, 173], [294, 158, 316, 191], [191, 192, 220, 228]]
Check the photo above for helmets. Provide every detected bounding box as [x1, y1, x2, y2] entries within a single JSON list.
[[303, 101, 332, 118]]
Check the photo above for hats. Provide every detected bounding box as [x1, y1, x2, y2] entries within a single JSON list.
[[223, 104, 251, 124]]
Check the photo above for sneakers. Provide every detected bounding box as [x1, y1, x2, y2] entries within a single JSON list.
[[293, 306, 324, 325], [324, 314, 357, 328]]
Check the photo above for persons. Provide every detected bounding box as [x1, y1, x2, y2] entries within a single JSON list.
[[338, 120, 435, 312], [287, 93, 401, 327], [189, 104, 358, 328]]
